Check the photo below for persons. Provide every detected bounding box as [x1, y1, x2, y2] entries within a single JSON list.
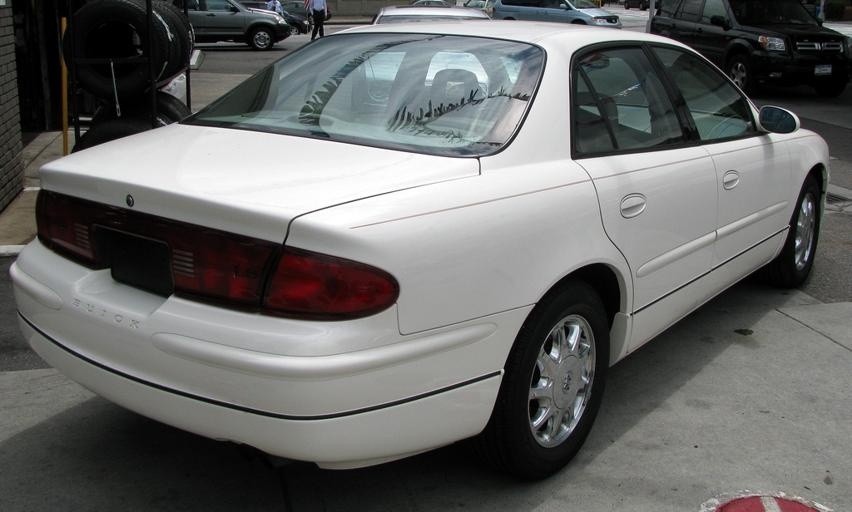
[[267, 0, 283, 17], [310, 0, 327, 40], [304, 0, 314, 31]]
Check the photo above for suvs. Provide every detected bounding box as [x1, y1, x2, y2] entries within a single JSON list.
[[644, 1, 851, 96], [168, 0, 292, 52]]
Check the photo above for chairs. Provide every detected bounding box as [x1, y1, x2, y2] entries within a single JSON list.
[[426, 66, 481, 117], [574, 90, 619, 142]]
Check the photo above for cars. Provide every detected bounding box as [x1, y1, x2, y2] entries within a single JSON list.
[[408, 1, 659, 32], [279, 1, 332, 24], [354, 4, 522, 113], [236, 2, 313, 35], [9, 24, 830, 475]]
